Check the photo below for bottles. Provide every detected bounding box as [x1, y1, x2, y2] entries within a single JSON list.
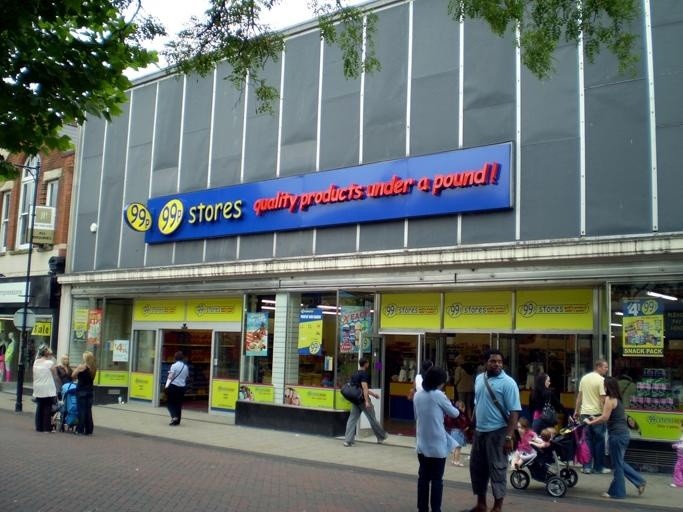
[[630, 378, 677, 410]]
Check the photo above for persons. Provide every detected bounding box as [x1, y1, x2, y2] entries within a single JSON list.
[[1, 331, 9, 383], [4, 332, 16, 383], [29, 342, 97, 437], [409, 353, 647, 512], [343, 356, 388, 446], [162, 352, 189, 427]]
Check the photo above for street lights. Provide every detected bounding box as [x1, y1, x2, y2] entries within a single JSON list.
[[11, 160, 42, 416]]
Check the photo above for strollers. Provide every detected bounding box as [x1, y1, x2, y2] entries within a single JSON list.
[[508, 415, 594, 499], [49, 370, 79, 432]]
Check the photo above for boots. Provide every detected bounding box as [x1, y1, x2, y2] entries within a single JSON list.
[[466, 496, 505, 512]]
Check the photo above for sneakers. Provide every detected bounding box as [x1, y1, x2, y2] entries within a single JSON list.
[[578, 466, 611, 475], [599, 480, 647, 501], [169, 416, 180, 426]]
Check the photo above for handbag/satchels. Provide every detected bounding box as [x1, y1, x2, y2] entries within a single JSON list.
[[340, 382, 366, 407]]
